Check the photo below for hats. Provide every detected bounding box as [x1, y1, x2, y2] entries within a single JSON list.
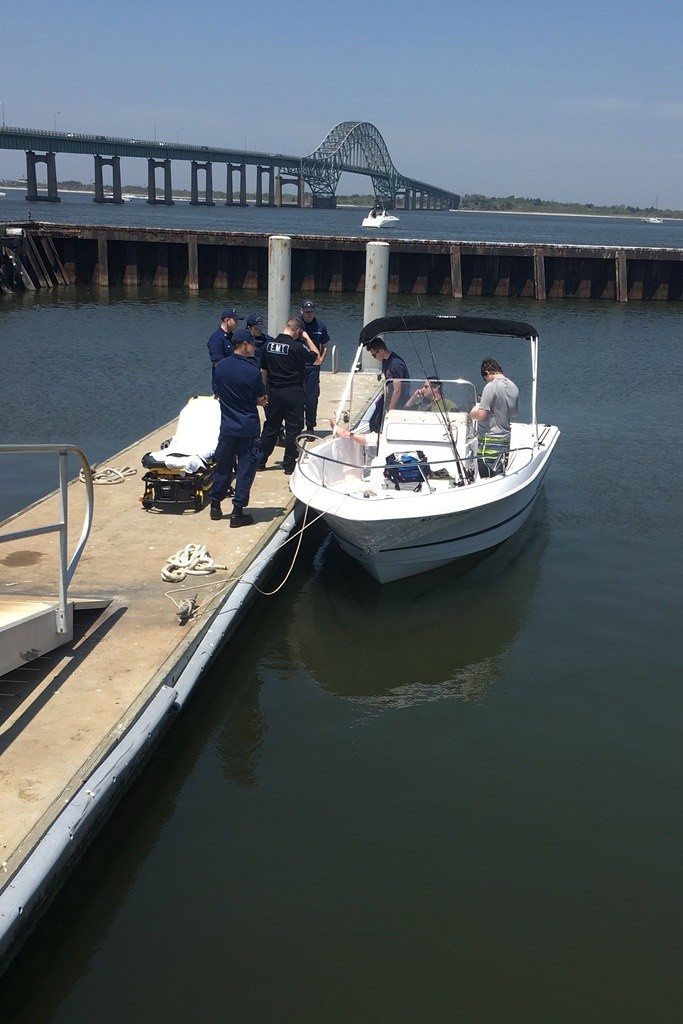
[[221, 308, 244, 320], [431, 468, 454, 480], [232, 329, 259, 343], [301, 299, 317, 311], [248, 315, 266, 329]]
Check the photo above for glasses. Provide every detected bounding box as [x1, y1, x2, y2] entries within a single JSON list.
[[299, 331, 302, 336], [425, 385, 427, 389], [306, 311, 313, 313], [483, 378, 486, 381], [372, 349, 380, 357]]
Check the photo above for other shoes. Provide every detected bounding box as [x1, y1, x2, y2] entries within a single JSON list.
[[285, 468, 292, 474], [307, 430, 315, 441]]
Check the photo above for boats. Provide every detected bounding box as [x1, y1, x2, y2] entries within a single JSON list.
[[642, 217, 664, 224], [292, 315, 561, 585], [361, 206, 400, 228]]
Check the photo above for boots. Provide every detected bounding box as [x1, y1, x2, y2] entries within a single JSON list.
[[231, 505, 253, 527], [210, 499, 222, 519]]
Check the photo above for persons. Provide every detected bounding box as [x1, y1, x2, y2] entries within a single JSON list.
[[207, 299, 331, 474], [469, 359, 519, 479], [331, 337, 460, 448], [373, 210, 388, 218], [208, 328, 269, 528]]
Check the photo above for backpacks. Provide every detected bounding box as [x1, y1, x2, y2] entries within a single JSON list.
[[384, 451, 430, 492]]
